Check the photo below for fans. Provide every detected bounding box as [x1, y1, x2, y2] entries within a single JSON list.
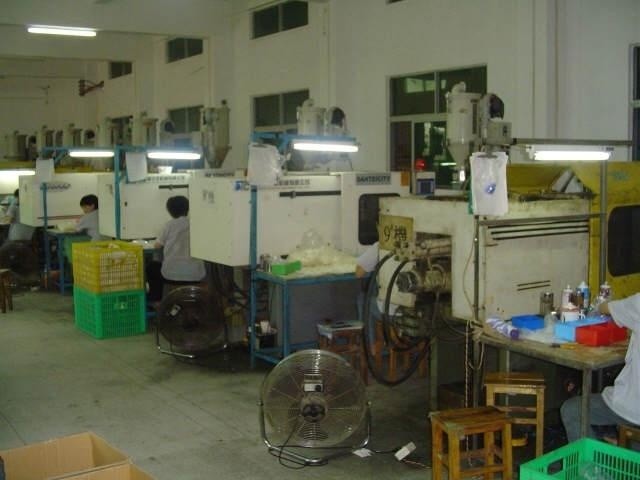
[[155, 285, 231, 358], [256, 349, 371, 469]]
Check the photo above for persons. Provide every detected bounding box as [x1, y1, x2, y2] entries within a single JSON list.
[[355, 238, 416, 357], [146, 196, 206, 304], [7, 189, 41, 293], [560, 291, 640, 444], [73, 194, 113, 241]]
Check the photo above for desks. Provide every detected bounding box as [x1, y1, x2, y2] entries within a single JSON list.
[[252, 257, 378, 380], [477, 303, 633, 439], [45, 226, 89, 295]]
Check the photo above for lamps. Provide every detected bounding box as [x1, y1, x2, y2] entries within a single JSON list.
[[534, 151, 610, 161], [88, 129, 95, 139], [292, 143, 358, 153], [489, 93, 504, 118], [165, 121, 172, 132], [331, 107, 344, 128], [32, 136, 36, 144]]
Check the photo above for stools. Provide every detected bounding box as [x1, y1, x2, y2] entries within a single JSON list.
[[618, 421, 640, 447], [317, 321, 368, 386], [0, 269, 13, 312], [376, 319, 427, 377], [483, 371, 544, 457], [127, 238, 165, 329], [429, 406, 512, 479]]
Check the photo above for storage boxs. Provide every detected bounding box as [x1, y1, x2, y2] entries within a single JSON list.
[[271, 260, 301, 275], [511, 314, 545, 330], [555, 319, 588, 342], [575, 325, 610, 346], [54, 462, 157, 480], [0, 430, 131, 480], [580, 318, 607, 325], [601, 323, 626, 342]]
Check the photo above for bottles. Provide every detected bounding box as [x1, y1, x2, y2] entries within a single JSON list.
[[540, 292, 554, 316], [576, 282, 589, 309], [487, 318, 519, 340], [562, 284, 574, 307], [600, 281, 611, 300]]
